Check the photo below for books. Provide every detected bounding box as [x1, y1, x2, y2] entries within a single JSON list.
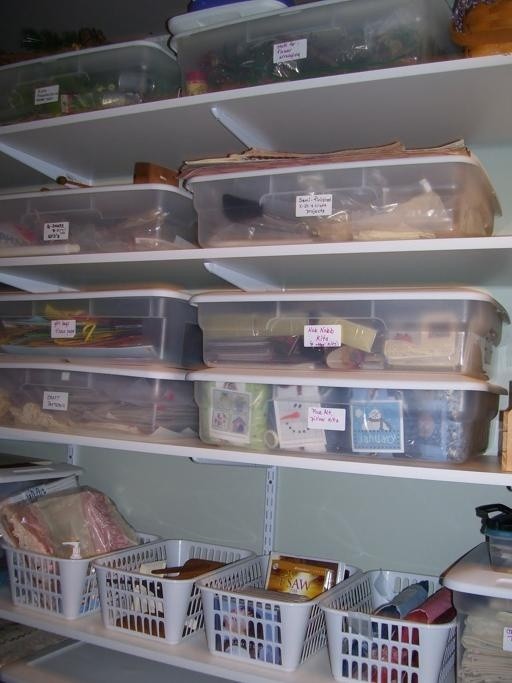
[[214, 552, 350, 667]]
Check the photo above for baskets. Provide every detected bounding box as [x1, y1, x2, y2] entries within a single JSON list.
[[90, 538, 257, 644], [2, 533, 159, 620], [195, 553, 363, 672], [317, 568, 460, 683], [2, 531, 455, 683]]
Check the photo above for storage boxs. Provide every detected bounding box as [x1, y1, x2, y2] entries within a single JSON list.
[[1, 364, 199, 439], [1, 184, 200, 257], [186, 373, 510, 465], [2, 290, 205, 370], [2, 39, 180, 127], [184, 155, 503, 247], [163, 2, 466, 100], [444, 538, 511, 683], [190, 288, 510, 380]]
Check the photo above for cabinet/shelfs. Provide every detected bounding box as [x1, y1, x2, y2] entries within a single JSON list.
[[0, 49, 509, 682]]
